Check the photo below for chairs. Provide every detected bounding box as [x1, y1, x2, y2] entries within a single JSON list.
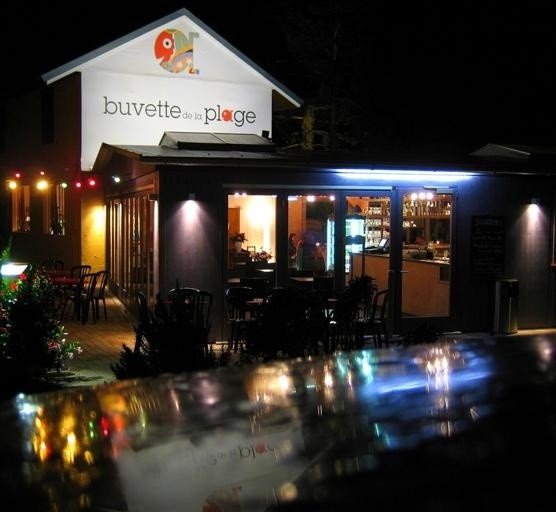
[[225, 276, 394, 364], [132, 286, 214, 367], [41, 260, 110, 326]]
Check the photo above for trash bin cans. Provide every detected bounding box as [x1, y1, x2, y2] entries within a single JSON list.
[[490, 279, 520, 334]]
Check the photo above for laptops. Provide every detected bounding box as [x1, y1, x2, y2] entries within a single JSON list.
[[365, 238, 389, 251]]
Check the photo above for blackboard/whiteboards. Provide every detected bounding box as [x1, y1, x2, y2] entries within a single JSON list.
[[462, 214, 506, 278]]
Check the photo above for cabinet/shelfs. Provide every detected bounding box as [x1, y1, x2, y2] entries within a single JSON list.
[[368, 198, 451, 248]]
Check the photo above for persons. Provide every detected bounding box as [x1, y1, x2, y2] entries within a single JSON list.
[[289, 231, 304, 259]]
